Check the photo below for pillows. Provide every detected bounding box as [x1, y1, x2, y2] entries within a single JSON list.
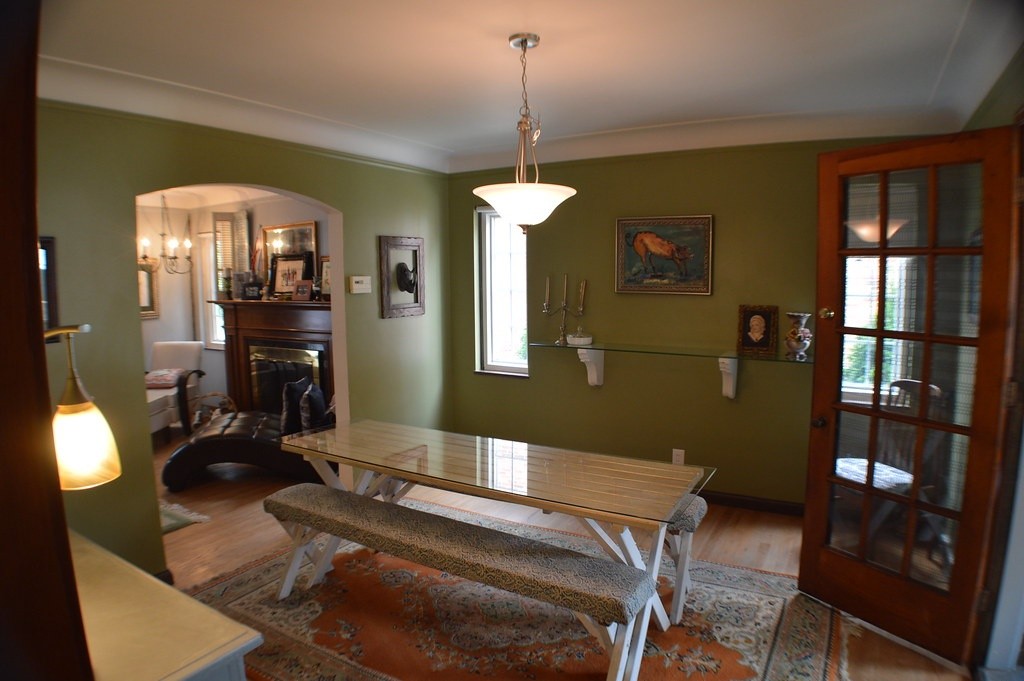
[[280, 375, 311, 437], [300, 380, 328, 431], [144, 366, 185, 388]]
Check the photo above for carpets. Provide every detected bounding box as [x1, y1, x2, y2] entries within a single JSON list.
[[183, 494, 861, 681]]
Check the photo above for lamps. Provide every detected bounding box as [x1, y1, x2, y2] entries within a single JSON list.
[[472, 35, 577, 233], [542, 272, 587, 345], [138, 193, 194, 275], [843, 182, 910, 242], [43, 321, 124, 490]]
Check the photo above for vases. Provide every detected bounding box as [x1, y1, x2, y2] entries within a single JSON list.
[[784, 312, 812, 361]]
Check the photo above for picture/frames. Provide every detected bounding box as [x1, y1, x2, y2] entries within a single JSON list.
[[737, 303, 779, 361], [242, 220, 331, 300], [614, 216, 711, 295], [379, 234, 426, 319], [137, 258, 160, 318]]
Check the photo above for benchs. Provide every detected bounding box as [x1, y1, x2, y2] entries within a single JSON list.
[[262, 485, 706, 662]]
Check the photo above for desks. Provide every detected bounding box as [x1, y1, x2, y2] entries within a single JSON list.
[[67, 521, 264, 681], [276, 421, 716, 681]]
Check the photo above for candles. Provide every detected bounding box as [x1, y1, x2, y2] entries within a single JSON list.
[[223, 266, 231, 277]]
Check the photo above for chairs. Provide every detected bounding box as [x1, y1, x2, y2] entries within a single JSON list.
[[835, 377, 942, 558], [161, 390, 340, 492], [145, 337, 205, 441]]
